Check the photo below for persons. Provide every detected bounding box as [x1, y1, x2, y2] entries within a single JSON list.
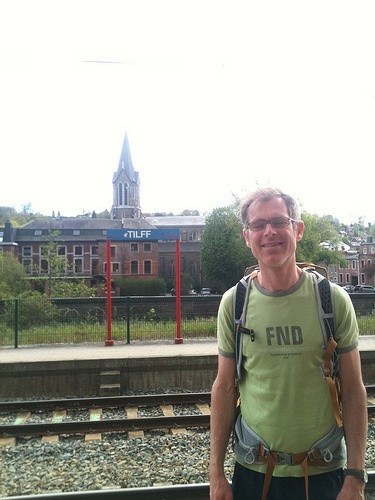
[[208, 191, 369, 500]]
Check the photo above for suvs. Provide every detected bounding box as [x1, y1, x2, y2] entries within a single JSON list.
[[354, 285, 375, 293]]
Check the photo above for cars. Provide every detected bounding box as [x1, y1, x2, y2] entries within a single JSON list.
[[342, 284, 355, 292], [188, 289, 197, 295], [201, 287, 211, 295]]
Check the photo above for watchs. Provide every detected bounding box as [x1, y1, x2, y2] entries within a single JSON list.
[[344, 468, 369, 483]]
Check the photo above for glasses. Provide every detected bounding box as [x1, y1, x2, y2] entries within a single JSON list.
[[246, 216, 296, 232]]
